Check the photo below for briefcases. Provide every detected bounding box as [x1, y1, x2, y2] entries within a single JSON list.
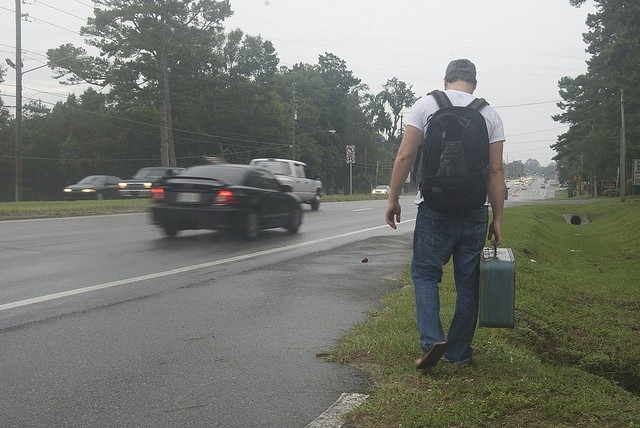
[[479, 241, 515, 328]]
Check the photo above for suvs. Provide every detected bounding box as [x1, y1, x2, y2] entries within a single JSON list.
[[249, 158, 322, 210], [117, 167, 185, 198]]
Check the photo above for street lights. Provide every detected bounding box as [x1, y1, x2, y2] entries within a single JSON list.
[[291, 130, 336, 160]]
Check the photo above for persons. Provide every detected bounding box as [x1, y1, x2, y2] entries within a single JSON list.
[[385, 59, 506, 368]]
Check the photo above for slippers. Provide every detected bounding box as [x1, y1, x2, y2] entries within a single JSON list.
[[450, 348, 473, 367], [414, 342, 447, 369]]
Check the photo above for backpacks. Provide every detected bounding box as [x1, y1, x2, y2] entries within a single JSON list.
[[413, 90, 490, 214]]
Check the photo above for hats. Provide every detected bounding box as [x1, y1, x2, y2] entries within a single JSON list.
[[445, 59, 476, 77]]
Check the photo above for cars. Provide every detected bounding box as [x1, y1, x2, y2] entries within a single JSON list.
[[63, 175, 121, 200], [372, 185, 390, 196], [150, 165, 302, 240]]
